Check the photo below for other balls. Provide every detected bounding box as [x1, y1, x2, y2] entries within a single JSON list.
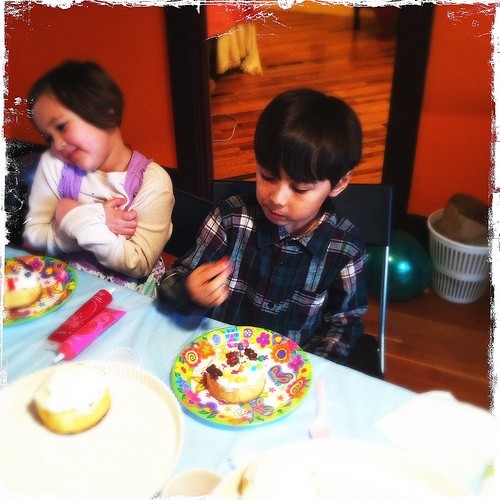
[[365, 231, 434, 299]]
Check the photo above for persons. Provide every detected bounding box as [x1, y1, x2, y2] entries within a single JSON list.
[[22, 61, 175, 297], [155, 87, 369, 368]]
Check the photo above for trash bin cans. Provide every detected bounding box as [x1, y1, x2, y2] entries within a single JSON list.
[[427, 208, 490, 303]]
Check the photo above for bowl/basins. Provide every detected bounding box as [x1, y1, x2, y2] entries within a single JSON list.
[[163, 469, 237, 500]]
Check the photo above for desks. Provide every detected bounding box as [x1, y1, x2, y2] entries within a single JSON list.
[[1, 246, 420, 475]]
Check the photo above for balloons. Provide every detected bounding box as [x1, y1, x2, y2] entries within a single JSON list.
[[367, 229, 432, 302]]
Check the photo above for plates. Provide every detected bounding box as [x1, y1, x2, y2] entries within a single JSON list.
[[170, 326, 313, 426], [0, 360, 184, 500], [2, 256, 77, 326]]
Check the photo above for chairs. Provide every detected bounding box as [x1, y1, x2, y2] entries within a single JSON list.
[[163, 181, 393, 380]]
